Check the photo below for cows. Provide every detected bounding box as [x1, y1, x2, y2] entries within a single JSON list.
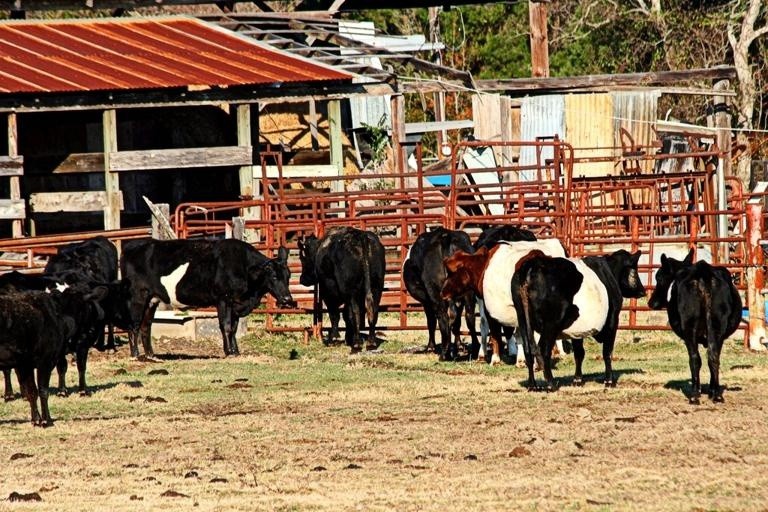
[[297, 226, 386, 354], [119, 237, 295, 364], [511, 249, 647, 392], [403, 224, 567, 371], [0, 235, 120, 429], [647, 248, 742, 405]]
